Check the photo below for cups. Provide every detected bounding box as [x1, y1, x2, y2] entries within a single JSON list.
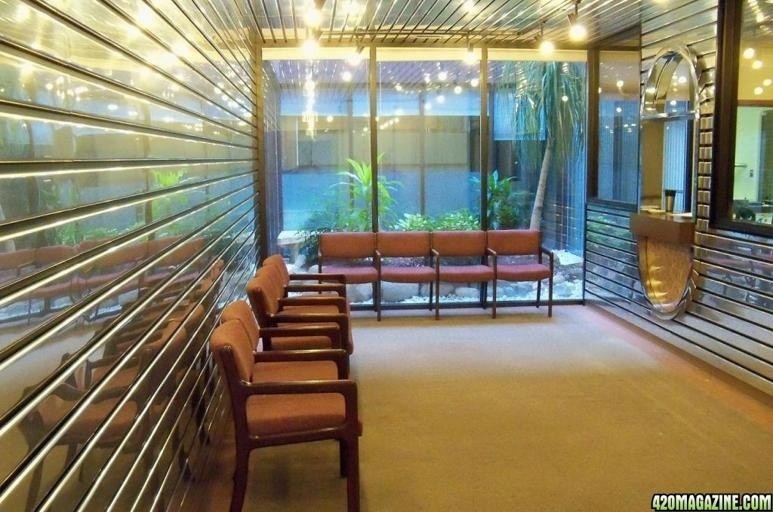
[[665, 189, 676, 212]]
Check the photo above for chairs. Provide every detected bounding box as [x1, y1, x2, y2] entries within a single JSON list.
[[207, 252, 365, 507], [317, 228, 556, 323]]
